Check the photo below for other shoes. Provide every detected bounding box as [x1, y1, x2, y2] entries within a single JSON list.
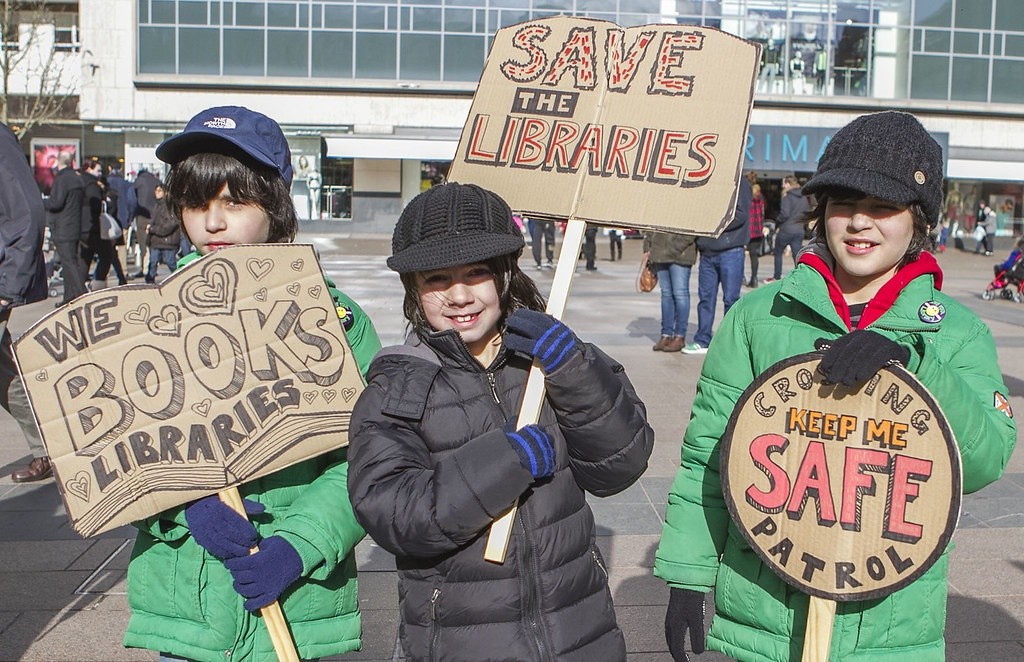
[[55, 301, 66, 308], [764, 277, 776, 283], [546, 261, 555, 268]]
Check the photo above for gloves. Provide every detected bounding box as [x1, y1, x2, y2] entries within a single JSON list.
[[504, 309, 583, 373], [816, 330, 909, 385], [185, 493, 266, 559], [224, 534, 304, 611], [506, 424, 558, 479], [665, 586, 706, 662]]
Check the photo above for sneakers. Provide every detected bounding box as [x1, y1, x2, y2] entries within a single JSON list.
[[680, 342, 708, 353]]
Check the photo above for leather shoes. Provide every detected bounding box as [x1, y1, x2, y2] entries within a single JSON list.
[[13, 457, 51, 483], [652, 336, 673, 350], [663, 336, 684, 352]]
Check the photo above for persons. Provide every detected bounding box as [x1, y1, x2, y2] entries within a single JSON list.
[[348, 181, 654, 662], [0, 121, 1024, 493], [648, 113, 1013, 662], [119, 105, 377, 662]]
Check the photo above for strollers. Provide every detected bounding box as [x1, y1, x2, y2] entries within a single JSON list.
[[982, 252, 1024, 300], [45, 257, 64, 298]]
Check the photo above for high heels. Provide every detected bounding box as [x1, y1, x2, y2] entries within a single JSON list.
[[747, 276, 758, 288]]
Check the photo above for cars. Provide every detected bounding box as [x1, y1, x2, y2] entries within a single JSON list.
[[624, 229, 644, 239]]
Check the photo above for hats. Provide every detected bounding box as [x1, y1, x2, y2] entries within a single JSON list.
[[387, 182, 528, 272], [801, 111, 943, 228], [155, 106, 293, 192]]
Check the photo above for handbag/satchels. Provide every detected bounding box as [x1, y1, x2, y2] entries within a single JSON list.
[[99, 201, 122, 240], [640, 261, 657, 292]]
